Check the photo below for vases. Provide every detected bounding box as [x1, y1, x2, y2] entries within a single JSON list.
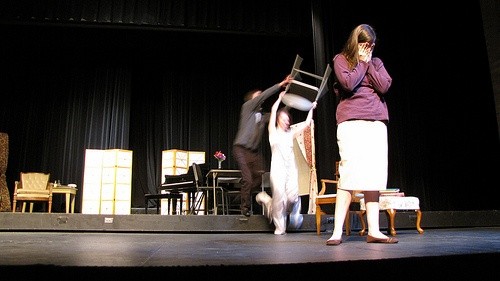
[[217, 160, 223, 169]]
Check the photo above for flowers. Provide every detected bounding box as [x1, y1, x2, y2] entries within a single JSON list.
[[214, 151, 226, 160]]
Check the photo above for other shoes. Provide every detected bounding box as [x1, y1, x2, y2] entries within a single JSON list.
[[367, 233, 398, 243], [243, 207, 249, 216], [327, 239, 342, 245]]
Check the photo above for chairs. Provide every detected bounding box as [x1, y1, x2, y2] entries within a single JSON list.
[[282, 54, 332, 111], [187, 163, 253, 215], [315, 162, 361, 236], [13, 172, 52, 213]]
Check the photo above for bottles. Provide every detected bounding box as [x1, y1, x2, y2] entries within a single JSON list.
[[54, 180, 61, 187]]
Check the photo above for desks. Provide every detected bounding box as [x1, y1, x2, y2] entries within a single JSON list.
[[359, 196, 424, 236], [22, 186, 78, 213], [206, 169, 265, 216]]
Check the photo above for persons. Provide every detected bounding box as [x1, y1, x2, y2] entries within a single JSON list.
[[323, 23, 399, 244], [256, 91, 318, 234], [231, 75, 292, 216]]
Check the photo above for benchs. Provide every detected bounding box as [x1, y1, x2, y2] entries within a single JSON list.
[[145, 194, 183, 215]]
